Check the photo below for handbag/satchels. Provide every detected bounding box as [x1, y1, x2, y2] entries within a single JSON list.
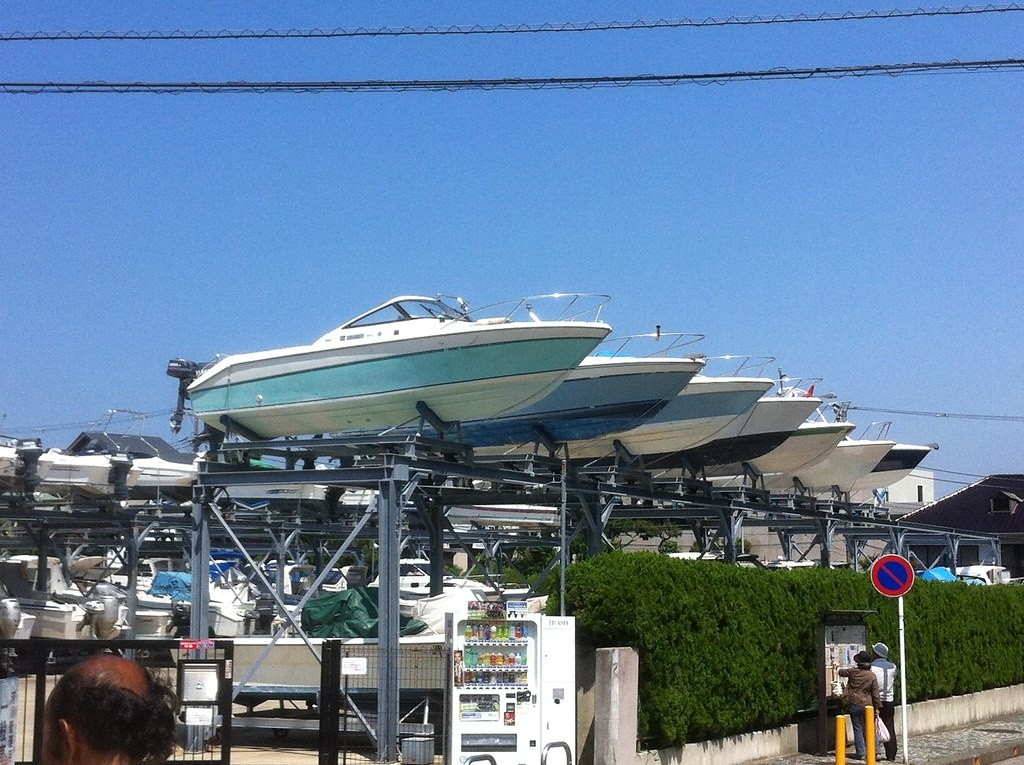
[[874, 715, 891, 742]]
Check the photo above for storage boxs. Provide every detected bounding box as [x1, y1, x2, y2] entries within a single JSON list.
[[401, 737, 435, 764]]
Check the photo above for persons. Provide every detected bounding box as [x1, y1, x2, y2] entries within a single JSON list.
[[869, 642, 897, 761], [832, 651, 886, 761], [41, 655, 181, 765]]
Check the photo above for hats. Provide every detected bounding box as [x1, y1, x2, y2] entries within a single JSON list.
[[853, 651, 871, 663], [872, 642, 889, 658]]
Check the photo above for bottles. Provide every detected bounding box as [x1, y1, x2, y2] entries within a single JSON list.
[[465, 624, 528, 642], [465, 649, 526, 668], [464, 669, 526, 683]]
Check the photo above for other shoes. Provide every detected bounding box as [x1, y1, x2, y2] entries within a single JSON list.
[[875, 753, 882, 760], [858, 754, 866, 760], [887, 756, 895, 761]]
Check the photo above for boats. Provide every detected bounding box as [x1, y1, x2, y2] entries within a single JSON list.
[[332, 325, 931, 510], [1, 432, 206, 515], [167, 292, 612, 445]]
